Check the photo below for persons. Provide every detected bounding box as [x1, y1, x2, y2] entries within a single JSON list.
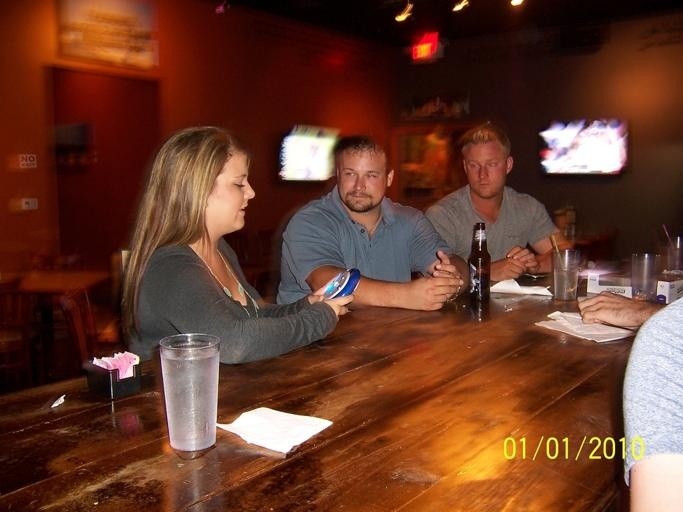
[[622, 298, 683, 512], [276, 136, 471, 311], [119, 126, 354, 365], [577, 291, 667, 331], [425, 126, 574, 282]]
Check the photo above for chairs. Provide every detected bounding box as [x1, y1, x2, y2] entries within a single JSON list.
[[56, 274, 126, 374]]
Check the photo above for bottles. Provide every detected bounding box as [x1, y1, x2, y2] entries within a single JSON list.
[[468, 222, 491, 316], [554, 204, 577, 226]]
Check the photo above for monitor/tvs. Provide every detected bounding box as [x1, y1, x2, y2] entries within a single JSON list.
[[538, 117, 630, 175], [277, 133, 337, 181]]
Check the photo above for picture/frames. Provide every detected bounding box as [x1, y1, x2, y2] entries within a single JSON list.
[[54, 0, 162, 72]]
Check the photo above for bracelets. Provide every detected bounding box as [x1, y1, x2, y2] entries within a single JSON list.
[[444, 282, 464, 305]]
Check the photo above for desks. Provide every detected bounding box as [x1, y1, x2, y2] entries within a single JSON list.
[[572, 227, 617, 261], [17, 263, 110, 294], [0, 272, 639, 512]]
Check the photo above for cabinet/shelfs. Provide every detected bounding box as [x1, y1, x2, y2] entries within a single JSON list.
[[392, 118, 487, 202]]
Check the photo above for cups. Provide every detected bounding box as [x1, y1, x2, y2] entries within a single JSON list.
[[666, 237, 683, 271], [552, 250, 580, 301], [631, 253, 659, 302], [159, 332, 222, 452]]
